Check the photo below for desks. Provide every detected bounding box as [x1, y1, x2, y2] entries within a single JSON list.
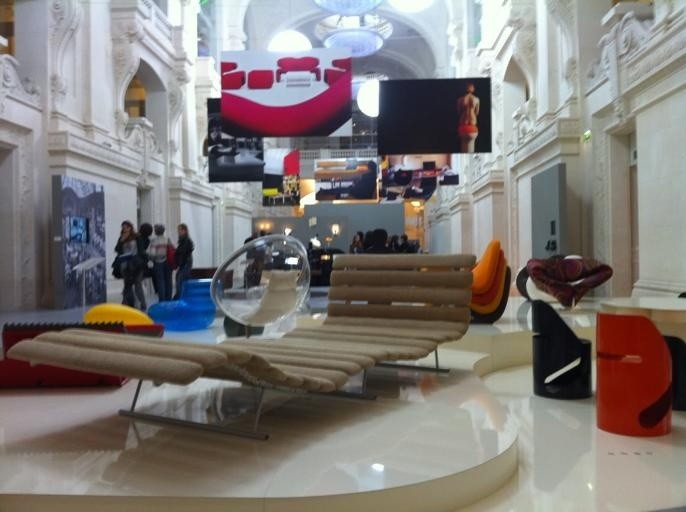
[[600, 296, 686, 411]]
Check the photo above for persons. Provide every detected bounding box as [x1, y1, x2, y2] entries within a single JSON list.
[[456, 82, 480, 152], [149, 222, 176, 302], [114, 220, 147, 311], [173, 222, 194, 300], [208, 118, 222, 146], [243, 231, 275, 270], [319, 160, 377, 198], [350, 228, 423, 254], [122, 222, 153, 305]]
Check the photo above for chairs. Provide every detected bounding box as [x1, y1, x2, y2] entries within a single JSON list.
[[469, 240, 512, 324], [148, 281, 221, 330], [5, 251, 477, 441], [516, 255, 613, 311], [596, 312, 671, 437], [532, 299, 593, 400]]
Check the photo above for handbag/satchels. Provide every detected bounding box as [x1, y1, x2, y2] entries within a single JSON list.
[[167, 245, 175, 265]]
